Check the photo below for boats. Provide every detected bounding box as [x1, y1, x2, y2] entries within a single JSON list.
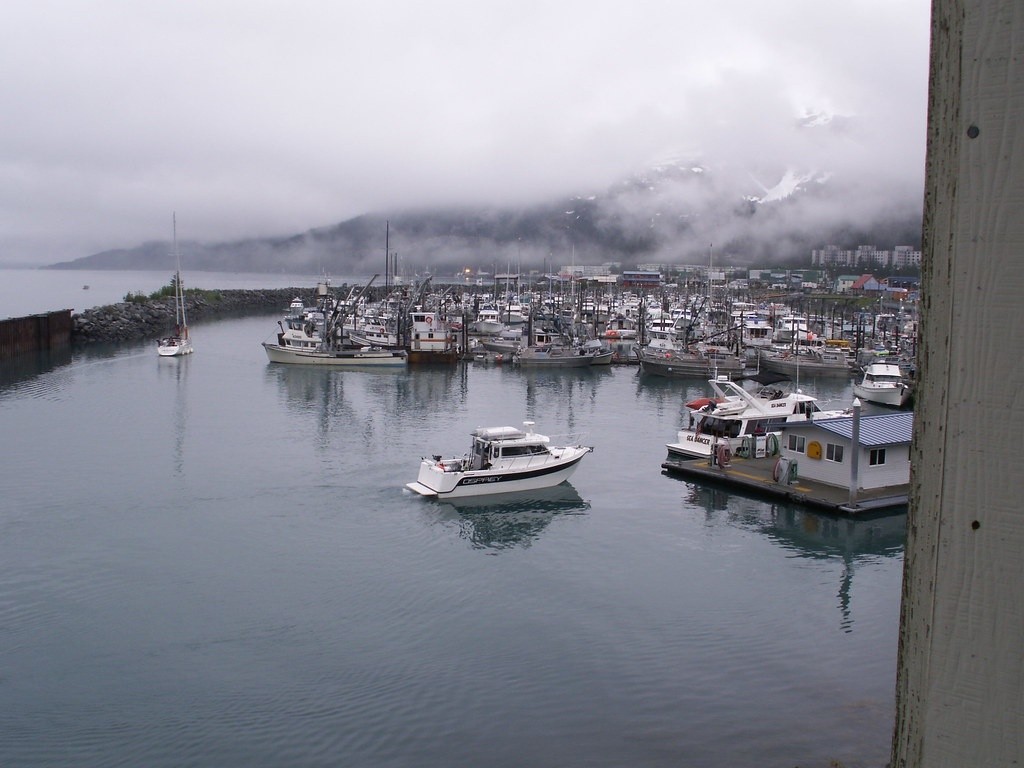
[[665, 367, 853, 459], [428, 480, 591, 553], [261, 221, 915, 378], [853, 364, 914, 406], [405, 421, 594, 499]]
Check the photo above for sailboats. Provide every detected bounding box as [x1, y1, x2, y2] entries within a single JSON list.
[[157, 212, 194, 356]]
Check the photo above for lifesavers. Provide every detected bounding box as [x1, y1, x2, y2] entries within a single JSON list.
[[426, 317, 432, 323], [380, 328, 385, 333]]
